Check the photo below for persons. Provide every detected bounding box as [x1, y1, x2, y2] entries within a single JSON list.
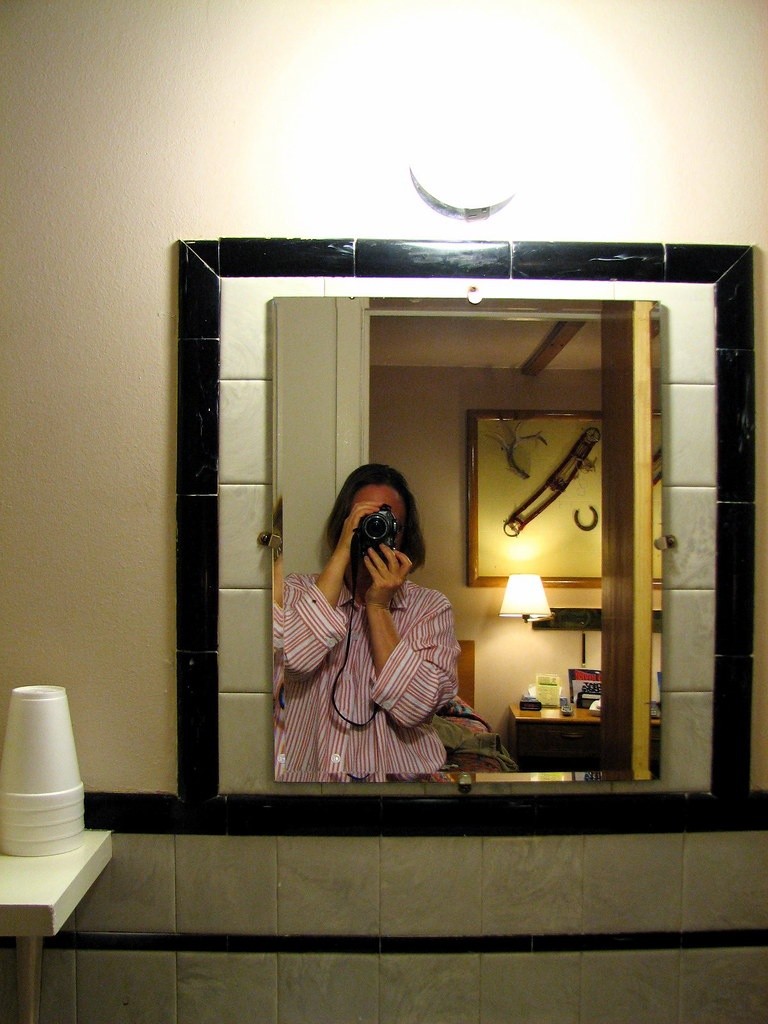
[[274, 463, 463, 784]]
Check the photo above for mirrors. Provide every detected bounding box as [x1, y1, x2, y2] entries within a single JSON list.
[[271, 296, 663, 783]]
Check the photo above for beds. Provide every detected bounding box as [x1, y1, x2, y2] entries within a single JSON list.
[[444, 641, 505, 771]]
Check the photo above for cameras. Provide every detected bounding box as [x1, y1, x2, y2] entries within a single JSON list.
[[357, 502, 398, 564]]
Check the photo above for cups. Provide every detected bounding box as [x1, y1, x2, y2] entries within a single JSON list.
[[0, 685, 85, 857]]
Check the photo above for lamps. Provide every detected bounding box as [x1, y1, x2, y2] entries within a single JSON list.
[[497, 573, 553, 623]]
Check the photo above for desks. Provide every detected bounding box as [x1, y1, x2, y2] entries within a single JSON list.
[[507, 704, 660, 772], [0, 831, 113, 1024]]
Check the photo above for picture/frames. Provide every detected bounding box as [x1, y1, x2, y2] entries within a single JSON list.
[[466, 409, 603, 589]]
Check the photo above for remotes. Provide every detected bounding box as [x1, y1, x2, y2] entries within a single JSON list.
[[561, 706, 573, 715]]
